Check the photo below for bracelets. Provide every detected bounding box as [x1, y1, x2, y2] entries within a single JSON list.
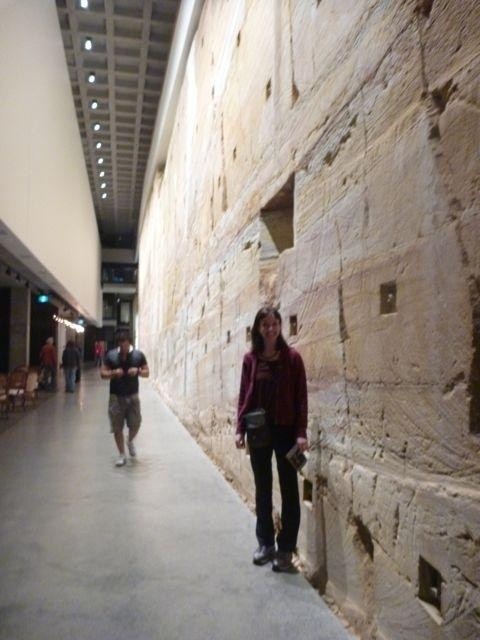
[[136, 366, 141, 376]]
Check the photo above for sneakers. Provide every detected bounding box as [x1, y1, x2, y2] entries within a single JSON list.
[[253, 543, 295, 570], [126, 439, 136, 457], [115, 454, 126, 466]]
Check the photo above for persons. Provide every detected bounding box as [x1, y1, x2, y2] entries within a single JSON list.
[[234, 306, 309, 570], [62, 337, 105, 394], [99, 328, 150, 467], [37, 336, 58, 390]]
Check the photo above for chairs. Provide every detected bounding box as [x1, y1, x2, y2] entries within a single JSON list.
[[0, 365, 41, 420]]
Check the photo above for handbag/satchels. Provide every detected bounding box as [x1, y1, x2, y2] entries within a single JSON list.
[[243, 409, 271, 452]]
